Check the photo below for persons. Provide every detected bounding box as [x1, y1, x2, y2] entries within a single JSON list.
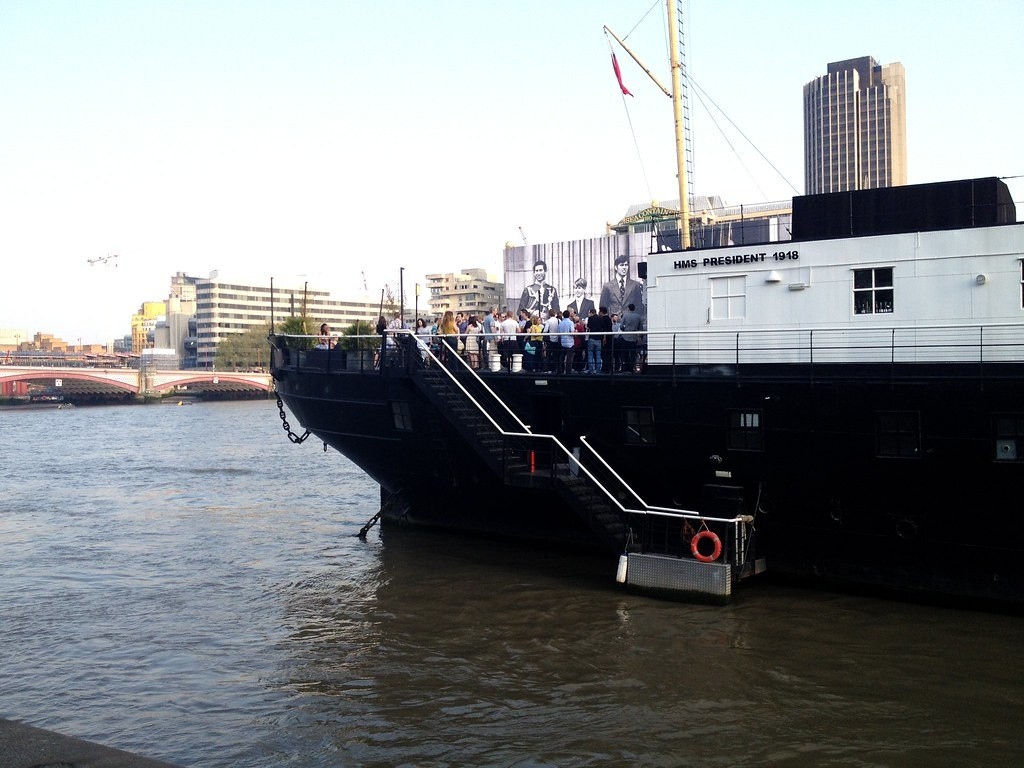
[[599, 256, 644, 323], [567, 278, 594, 318], [315, 324, 338, 349], [516, 261, 560, 320], [374, 304, 647, 373]]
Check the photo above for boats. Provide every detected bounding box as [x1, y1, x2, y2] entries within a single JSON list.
[[266, 1, 1023, 618]]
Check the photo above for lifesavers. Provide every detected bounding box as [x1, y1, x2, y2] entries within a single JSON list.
[[690, 531, 722, 562]]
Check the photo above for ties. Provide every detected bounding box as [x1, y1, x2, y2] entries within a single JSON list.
[[619, 279, 625, 302]]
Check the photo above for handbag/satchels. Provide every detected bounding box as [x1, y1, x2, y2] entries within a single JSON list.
[[477, 325, 485, 342], [457, 335, 464, 351], [524, 341, 536, 356]]
